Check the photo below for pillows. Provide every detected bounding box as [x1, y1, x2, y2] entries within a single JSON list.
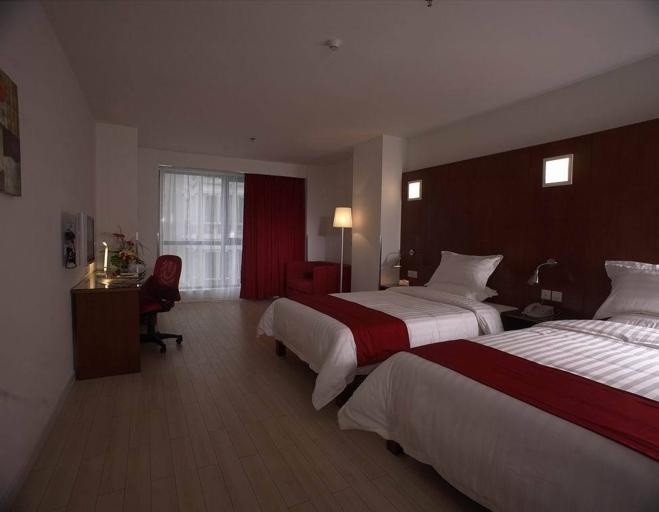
[[609, 313, 659, 330], [472, 287, 498, 303], [424, 250, 503, 297], [592, 260, 659, 320]]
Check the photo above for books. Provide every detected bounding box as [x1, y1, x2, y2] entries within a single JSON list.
[[120, 272, 145, 278]]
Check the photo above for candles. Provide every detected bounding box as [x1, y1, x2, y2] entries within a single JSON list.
[[103, 242, 108, 268], [135, 232, 138, 240]]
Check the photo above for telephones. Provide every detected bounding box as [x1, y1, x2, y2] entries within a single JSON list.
[[522, 303, 554, 319]]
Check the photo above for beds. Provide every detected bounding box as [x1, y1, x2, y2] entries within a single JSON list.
[[255, 285, 520, 412], [337, 318, 659, 512]]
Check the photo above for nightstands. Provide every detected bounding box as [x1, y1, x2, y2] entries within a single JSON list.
[[501, 310, 572, 330], [379, 284, 415, 291]]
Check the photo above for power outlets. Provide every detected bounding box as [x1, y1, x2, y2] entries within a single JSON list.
[[540, 289, 552, 301], [552, 291, 562, 302]]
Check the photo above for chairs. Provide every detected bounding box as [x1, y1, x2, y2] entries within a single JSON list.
[[139, 254, 182, 353], [286, 261, 351, 298]]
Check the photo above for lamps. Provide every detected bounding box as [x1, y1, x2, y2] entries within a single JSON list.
[[332, 207, 352, 293], [393, 249, 415, 268], [527, 259, 559, 287]]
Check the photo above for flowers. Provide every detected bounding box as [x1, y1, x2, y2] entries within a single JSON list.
[[103, 225, 145, 272]]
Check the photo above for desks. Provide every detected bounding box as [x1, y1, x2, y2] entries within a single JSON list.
[[70, 270, 144, 380]]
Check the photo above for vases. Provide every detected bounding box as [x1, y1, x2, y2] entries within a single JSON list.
[[127, 263, 138, 273]]
